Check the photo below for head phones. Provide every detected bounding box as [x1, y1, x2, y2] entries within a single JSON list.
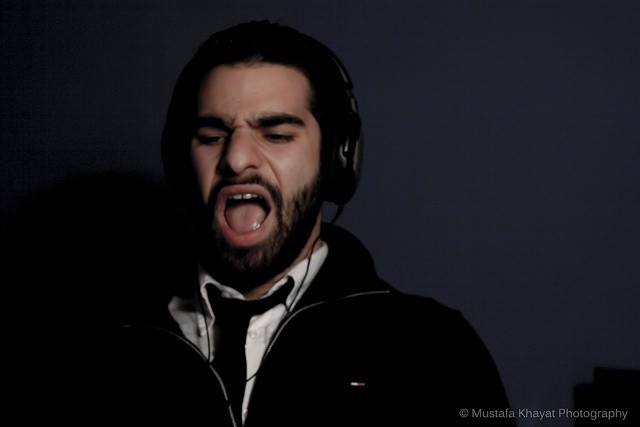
[[160, 21, 364, 205]]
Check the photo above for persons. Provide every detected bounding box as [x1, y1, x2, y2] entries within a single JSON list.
[[44, 24, 518, 427]]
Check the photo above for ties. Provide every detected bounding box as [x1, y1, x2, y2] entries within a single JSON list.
[[205, 277, 295, 427]]
